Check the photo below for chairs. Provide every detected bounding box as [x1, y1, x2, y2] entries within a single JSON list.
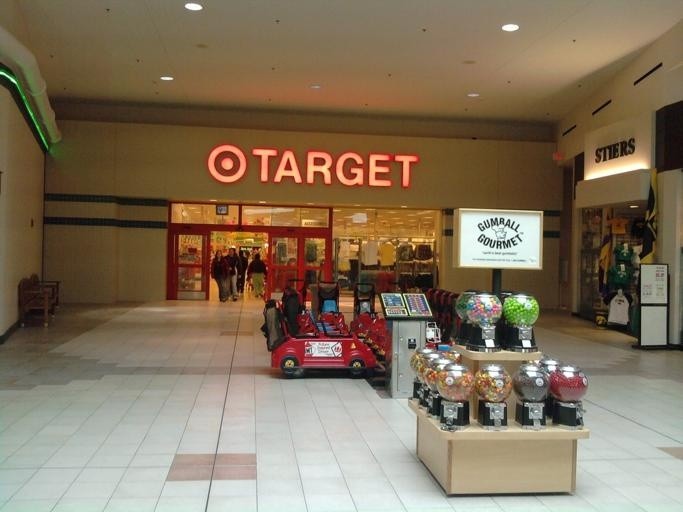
[[18, 274, 62, 328]]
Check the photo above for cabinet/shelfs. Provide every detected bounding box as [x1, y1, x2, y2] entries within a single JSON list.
[[408, 345, 590, 497]]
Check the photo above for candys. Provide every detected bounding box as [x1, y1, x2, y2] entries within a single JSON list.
[[411, 293, 587, 403]]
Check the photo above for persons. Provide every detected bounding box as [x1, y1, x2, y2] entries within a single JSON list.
[[211, 248, 248, 302], [246, 253, 267, 298]]
